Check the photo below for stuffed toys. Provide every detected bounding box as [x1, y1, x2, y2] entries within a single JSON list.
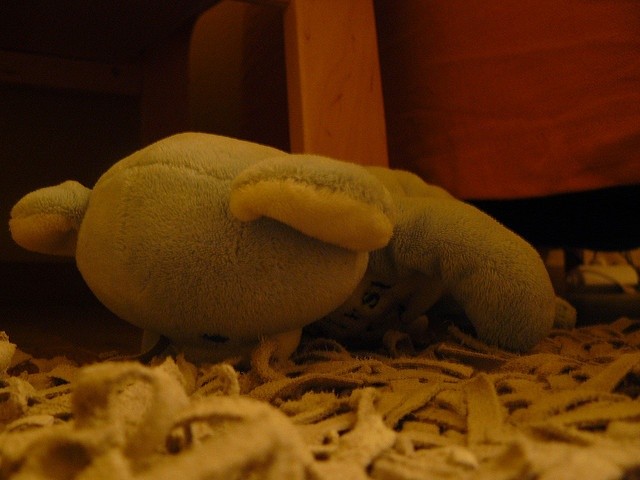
[[7, 131, 579, 368]]
